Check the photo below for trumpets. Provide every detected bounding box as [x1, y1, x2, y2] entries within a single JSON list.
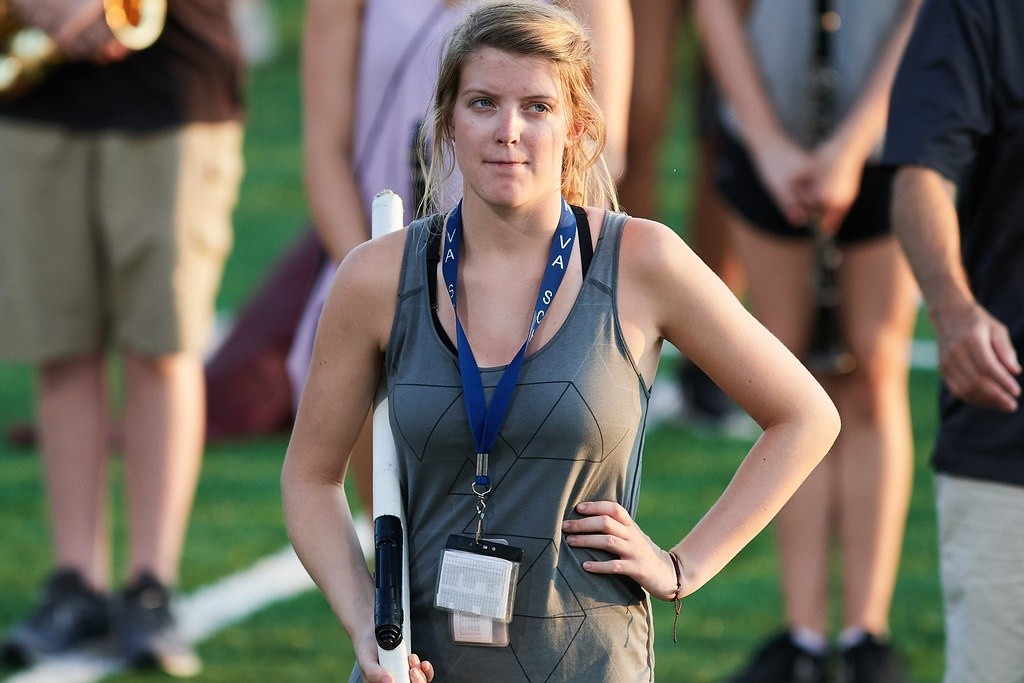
[[0, 0, 168, 95]]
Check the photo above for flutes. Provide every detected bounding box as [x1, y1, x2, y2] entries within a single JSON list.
[[795, 0, 859, 381]]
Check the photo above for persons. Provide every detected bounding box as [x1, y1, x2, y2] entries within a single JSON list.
[[619, 2, 755, 423], [252, 2, 845, 683], [689, 2, 933, 683], [1, 0, 250, 679], [293, 0, 650, 683], [881, 0, 1024, 683]]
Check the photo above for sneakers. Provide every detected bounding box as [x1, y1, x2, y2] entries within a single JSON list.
[[725, 621, 832, 683], [843, 627, 908, 683], [107, 568, 202, 679], [2, 565, 116, 669]]
